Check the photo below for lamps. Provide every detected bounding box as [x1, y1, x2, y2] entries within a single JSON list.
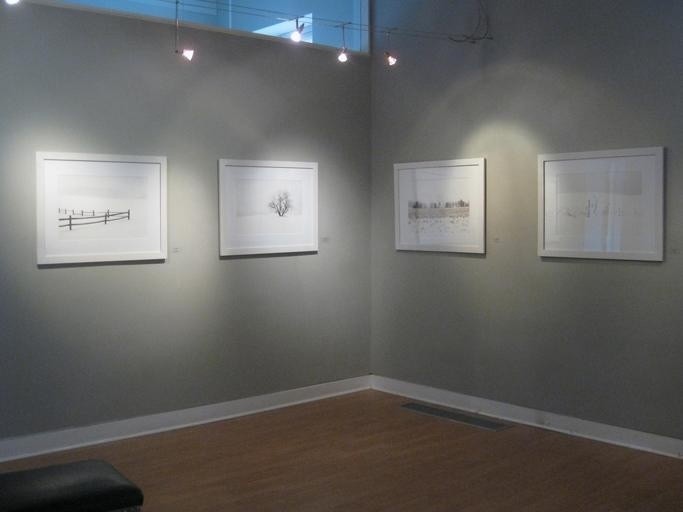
[[338, 25, 348, 64], [291, 17, 305, 44], [384, 33, 397, 66], [172, 3, 194, 62]]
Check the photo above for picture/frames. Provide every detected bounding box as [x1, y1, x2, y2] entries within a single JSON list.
[[538, 145, 665, 261], [218, 157, 318, 257], [35, 151, 168, 263], [394, 157, 485, 256]]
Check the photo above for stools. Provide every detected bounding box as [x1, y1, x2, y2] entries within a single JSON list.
[[1, 458, 144, 511]]
